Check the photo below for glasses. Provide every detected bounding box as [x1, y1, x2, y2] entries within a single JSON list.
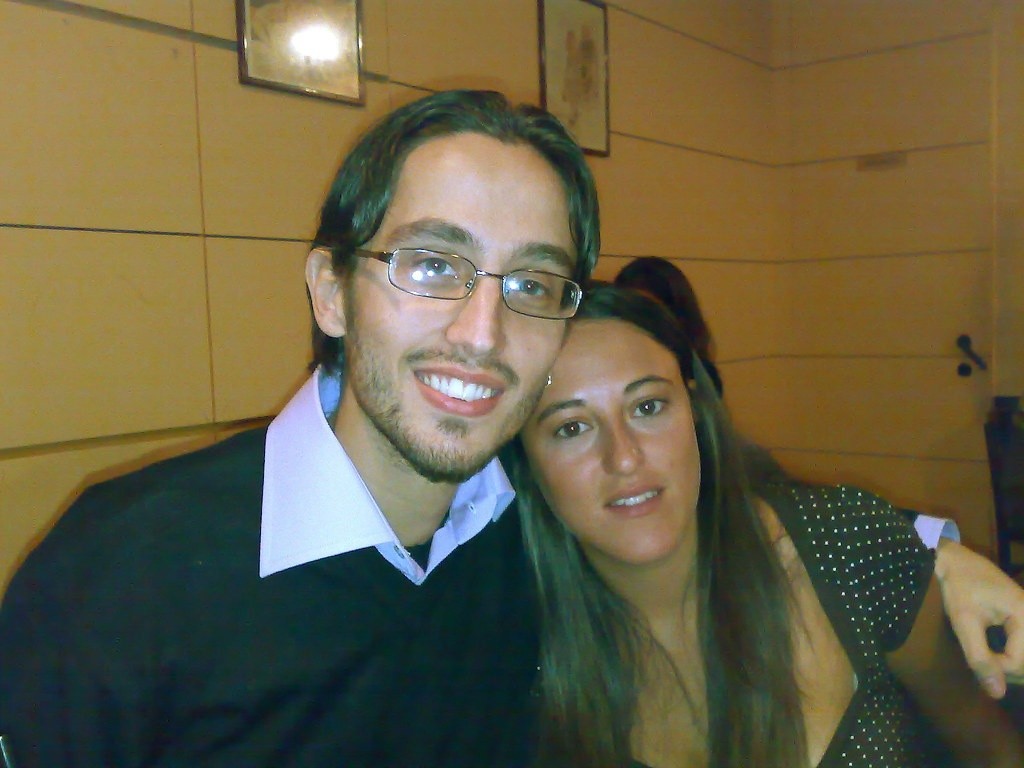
[[347, 247, 582, 319]]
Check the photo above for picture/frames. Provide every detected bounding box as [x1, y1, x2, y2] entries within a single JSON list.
[[236, 0, 367, 108], [535, 0, 611, 157]]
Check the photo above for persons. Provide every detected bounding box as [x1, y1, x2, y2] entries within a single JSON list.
[[0, 90, 1023, 768], [508, 277, 1024, 768], [611, 255, 726, 400]]
